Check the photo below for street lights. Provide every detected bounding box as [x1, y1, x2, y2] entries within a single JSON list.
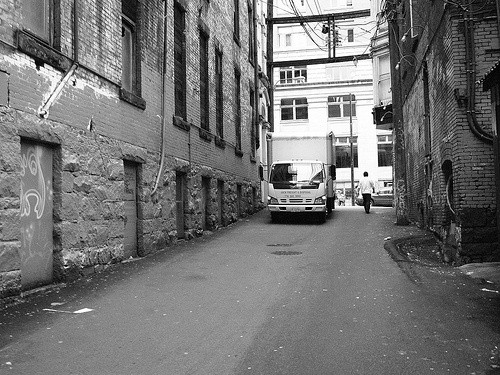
[[273, 75, 305, 88]]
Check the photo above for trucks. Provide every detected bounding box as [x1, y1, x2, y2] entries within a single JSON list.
[[259, 130, 337, 223]]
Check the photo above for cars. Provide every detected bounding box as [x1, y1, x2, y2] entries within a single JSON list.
[[356, 187, 395, 207]]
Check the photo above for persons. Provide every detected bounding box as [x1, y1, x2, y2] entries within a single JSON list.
[[357, 172, 375, 213]]
